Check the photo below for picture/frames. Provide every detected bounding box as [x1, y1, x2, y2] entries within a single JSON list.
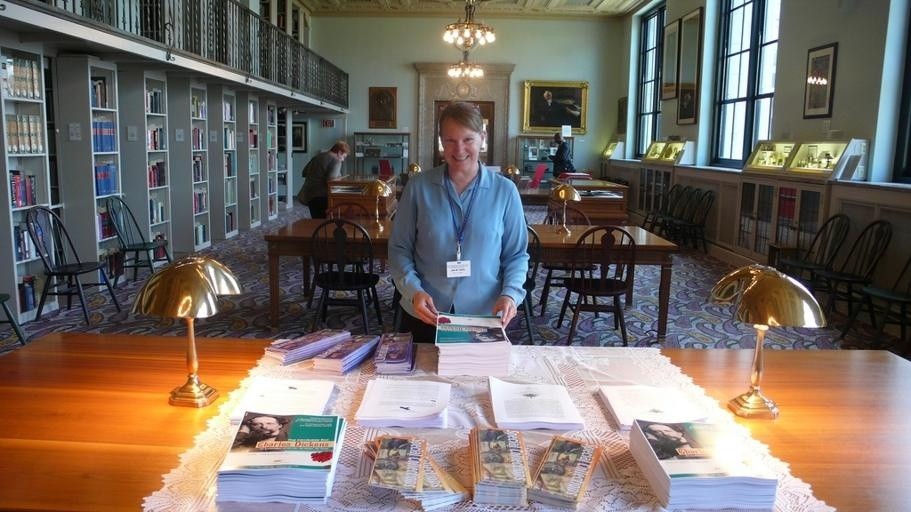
[[522, 80, 589, 135], [660, 6, 703, 125], [277, 121, 307, 153], [802, 42, 838, 119]]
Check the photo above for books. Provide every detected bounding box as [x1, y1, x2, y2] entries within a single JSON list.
[[599, 385, 675, 429], [231, 376, 336, 422], [488, 375, 583, 431], [249, 101, 259, 222], [146, 88, 168, 259], [192, 95, 208, 245], [630, 420, 777, 512], [361, 427, 604, 512], [223, 101, 237, 234], [435, 310, 512, 377], [267, 105, 275, 216], [265, 328, 413, 376], [214, 411, 346, 509], [354, 379, 452, 429], [90, 76, 124, 283], [1, 57, 47, 309]]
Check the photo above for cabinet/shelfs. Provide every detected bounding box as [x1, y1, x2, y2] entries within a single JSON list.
[[353, 132, 411, 186], [174, 79, 213, 253], [55, 54, 121, 284], [733, 176, 826, 269], [637, 166, 673, 222], [1, 34, 61, 325], [123, 65, 172, 268], [249, 95, 261, 228], [221, 87, 238, 238], [267, 101, 279, 222], [515, 136, 574, 176]]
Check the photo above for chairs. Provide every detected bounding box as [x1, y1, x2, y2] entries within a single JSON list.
[[779, 214, 911, 357], [310, 219, 383, 331], [518, 225, 540, 344], [306, 204, 375, 304], [539, 208, 600, 319], [641, 183, 716, 253], [558, 226, 635, 342]]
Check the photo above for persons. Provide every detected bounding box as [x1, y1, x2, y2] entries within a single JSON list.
[[299, 141, 351, 218], [540, 91, 565, 123], [551, 132, 572, 178], [396, 102, 530, 341]]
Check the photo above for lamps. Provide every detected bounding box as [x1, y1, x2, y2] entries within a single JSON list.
[[443, 0, 496, 46], [129, 256, 244, 407], [503, 164, 520, 180], [447, 46, 484, 79], [406, 162, 422, 176], [705, 263, 827, 420]]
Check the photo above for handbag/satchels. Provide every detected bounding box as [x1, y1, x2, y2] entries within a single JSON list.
[[297, 180, 307, 206]]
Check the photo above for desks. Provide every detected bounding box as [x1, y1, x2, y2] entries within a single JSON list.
[[268, 218, 678, 344], [323, 174, 630, 221], [0, 333, 911, 512]]
